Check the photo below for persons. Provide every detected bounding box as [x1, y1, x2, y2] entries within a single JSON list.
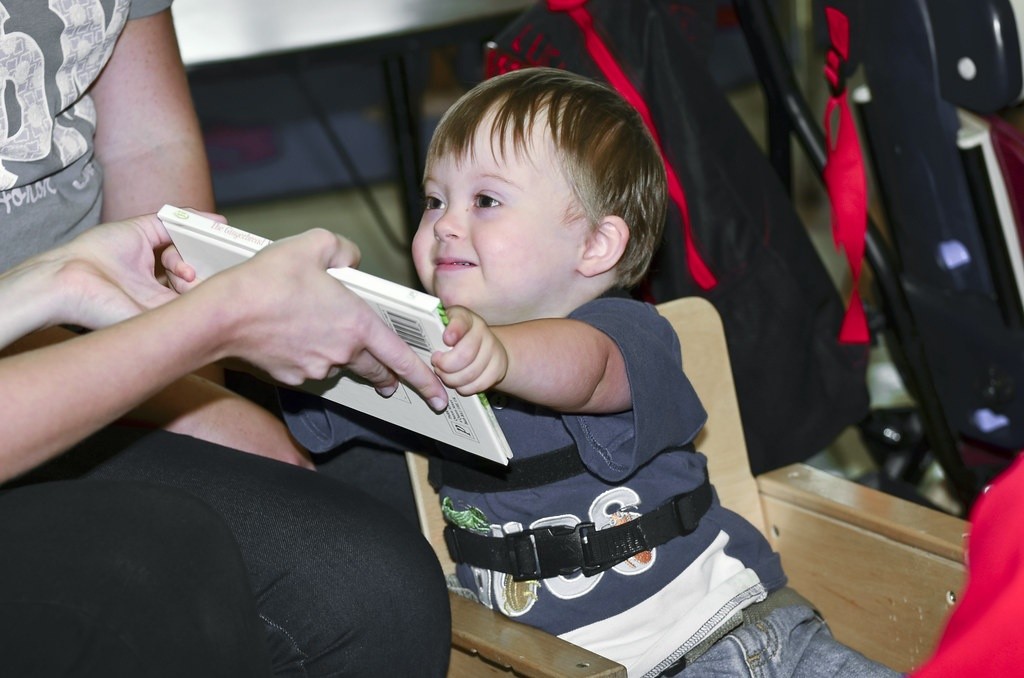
[[0, 199, 457, 678], [0, 0, 225, 368], [158, 66, 911, 677]]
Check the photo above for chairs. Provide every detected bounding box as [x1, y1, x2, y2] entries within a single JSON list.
[[395, 295, 970, 678]]
